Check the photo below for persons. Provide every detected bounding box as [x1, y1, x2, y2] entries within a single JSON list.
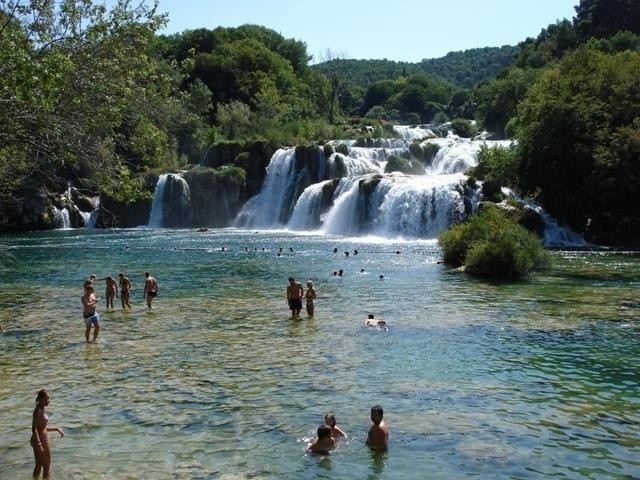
[[360, 269, 365, 272], [222, 247, 294, 257], [80, 270, 133, 343], [325, 413, 345, 438], [332, 248, 358, 256], [380, 274, 383, 280], [287, 277, 304, 316], [333, 269, 344, 280], [29, 389, 65, 479], [143, 271, 159, 311], [366, 405, 389, 452], [304, 280, 316, 317], [309, 424, 335, 452], [365, 314, 388, 332]]
